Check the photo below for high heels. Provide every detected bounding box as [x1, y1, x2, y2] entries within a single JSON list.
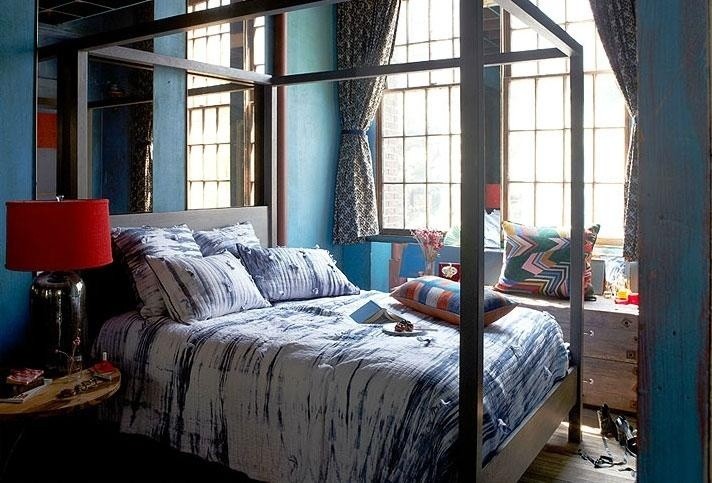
[[596, 404, 638, 456]]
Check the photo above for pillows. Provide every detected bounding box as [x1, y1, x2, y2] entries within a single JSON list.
[[492, 220, 600, 299], [236, 243, 360, 302], [192, 221, 261, 259], [111, 223, 204, 319], [389, 276, 519, 328], [145, 248, 273, 325]]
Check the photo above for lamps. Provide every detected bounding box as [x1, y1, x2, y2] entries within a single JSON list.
[[6, 199, 113, 378]]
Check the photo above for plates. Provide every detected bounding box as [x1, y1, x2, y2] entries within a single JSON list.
[[382, 322, 423, 334]]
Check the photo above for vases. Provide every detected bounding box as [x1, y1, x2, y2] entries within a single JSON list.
[[425, 262, 434, 276]]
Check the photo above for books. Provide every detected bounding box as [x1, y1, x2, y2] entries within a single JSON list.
[[0, 368, 53, 404], [349, 299, 405, 324]]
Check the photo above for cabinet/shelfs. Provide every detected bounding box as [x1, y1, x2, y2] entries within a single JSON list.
[[487, 285, 638, 417]]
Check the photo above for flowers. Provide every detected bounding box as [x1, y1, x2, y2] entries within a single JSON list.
[[410, 228, 444, 274]]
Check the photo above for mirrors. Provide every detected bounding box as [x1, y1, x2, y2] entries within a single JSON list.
[[34, 0, 267, 217]]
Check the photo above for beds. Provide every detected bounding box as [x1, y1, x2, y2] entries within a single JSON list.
[[56, 0, 584, 479]]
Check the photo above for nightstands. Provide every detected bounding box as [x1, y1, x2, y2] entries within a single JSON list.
[[0, 363, 122, 479]]
